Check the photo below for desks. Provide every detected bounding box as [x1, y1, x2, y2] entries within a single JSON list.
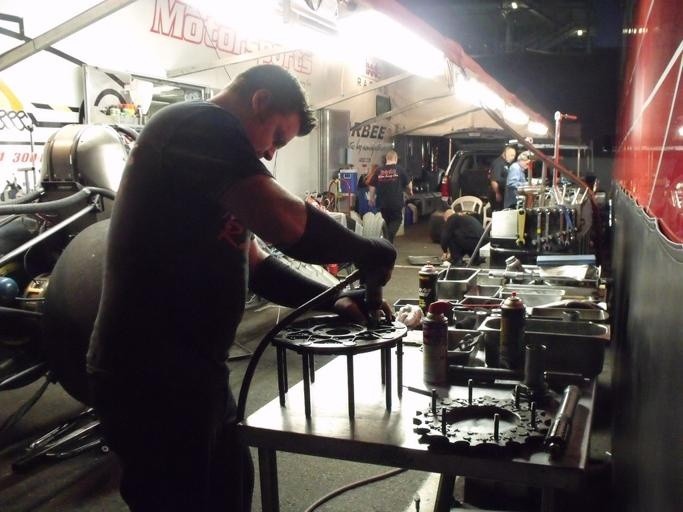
[[237, 326, 598, 510]]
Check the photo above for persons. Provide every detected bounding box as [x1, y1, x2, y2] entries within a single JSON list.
[[367, 148, 413, 245], [441, 208, 488, 268], [503, 151, 534, 209], [486, 147, 516, 210], [85, 62, 398, 512]]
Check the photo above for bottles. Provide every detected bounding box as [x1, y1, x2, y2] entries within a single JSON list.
[[418, 260, 439, 317], [499, 291, 526, 369], [505, 256, 525, 272], [421, 301, 453, 385], [109, 103, 143, 126]]
